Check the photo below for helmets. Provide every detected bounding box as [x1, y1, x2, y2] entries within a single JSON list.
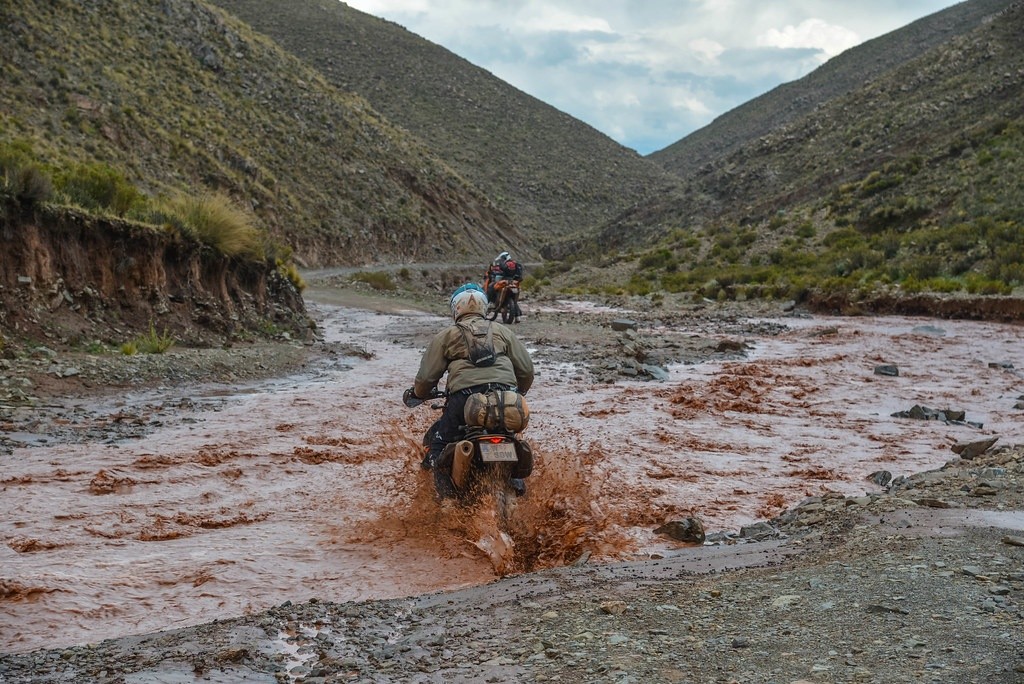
[[495, 252, 511, 263], [450, 283, 489, 323]]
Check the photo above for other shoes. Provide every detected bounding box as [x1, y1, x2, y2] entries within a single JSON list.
[[515, 316, 521, 323], [488, 302, 495, 309]]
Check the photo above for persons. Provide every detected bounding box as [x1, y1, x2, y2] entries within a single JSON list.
[[415, 283, 535, 467], [494, 252, 527, 324]]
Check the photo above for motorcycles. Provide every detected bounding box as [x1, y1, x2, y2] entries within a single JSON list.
[[406, 383, 534, 534], [481, 272, 520, 325]]
